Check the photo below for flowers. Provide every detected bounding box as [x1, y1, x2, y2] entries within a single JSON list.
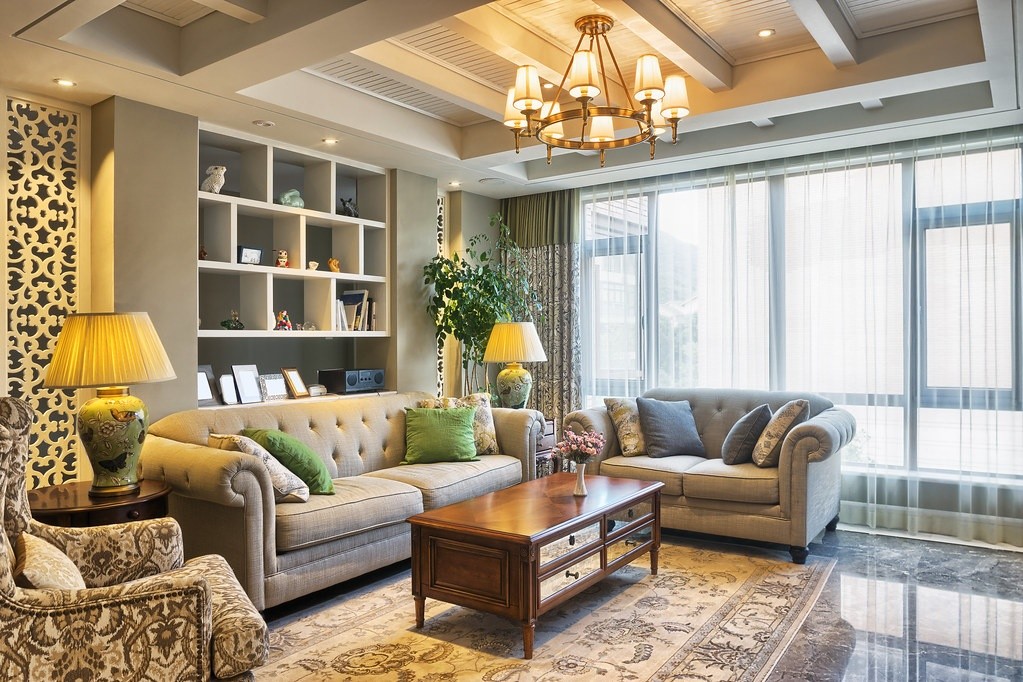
[[549, 425, 608, 464]]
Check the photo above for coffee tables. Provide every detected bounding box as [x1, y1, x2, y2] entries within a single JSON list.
[[404, 471, 667, 659]]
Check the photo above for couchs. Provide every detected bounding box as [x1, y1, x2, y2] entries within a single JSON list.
[[139, 391, 546, 616], [559, 385, 857, 566], [0, 395, 268, 681]]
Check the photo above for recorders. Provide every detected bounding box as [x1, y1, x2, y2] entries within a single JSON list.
[[317, 370, 385, 394]]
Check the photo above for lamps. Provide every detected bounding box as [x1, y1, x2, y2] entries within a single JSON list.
[[43, 310, 178, 499], [483, 322, 549, 410], [503, 13, 689, 166]]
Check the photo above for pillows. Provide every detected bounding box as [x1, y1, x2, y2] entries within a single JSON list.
[[604, 396, 656, 457], [636, 398, 706, 457], [417, 393, 500, 456], [209, 433, 311, 503], [753, 398, 811, 467], [721, 403, 773, 465], [403, 406, 478, 466], [13, 533, 90, 593], [242, 428, 336, 496]]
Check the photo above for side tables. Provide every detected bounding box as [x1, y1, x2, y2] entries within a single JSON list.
[[535, 416, 561, 474], [27, 480, 176, 530]]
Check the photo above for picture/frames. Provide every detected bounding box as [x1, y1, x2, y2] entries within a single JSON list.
[[219, 374, 239, 403], [231, 363, 263, 404], [198, 364, 223, 404], [281, 367, 308, 397], [260, 374, 289, 400]]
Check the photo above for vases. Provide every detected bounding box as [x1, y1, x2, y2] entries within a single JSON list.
[[573, 462, 587, 497]]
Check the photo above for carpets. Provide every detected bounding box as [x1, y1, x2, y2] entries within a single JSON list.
[[243, 542, 839, 682]]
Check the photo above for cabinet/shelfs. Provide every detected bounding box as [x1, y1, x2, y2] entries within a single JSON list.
[[195, 123, 402, 405]]
